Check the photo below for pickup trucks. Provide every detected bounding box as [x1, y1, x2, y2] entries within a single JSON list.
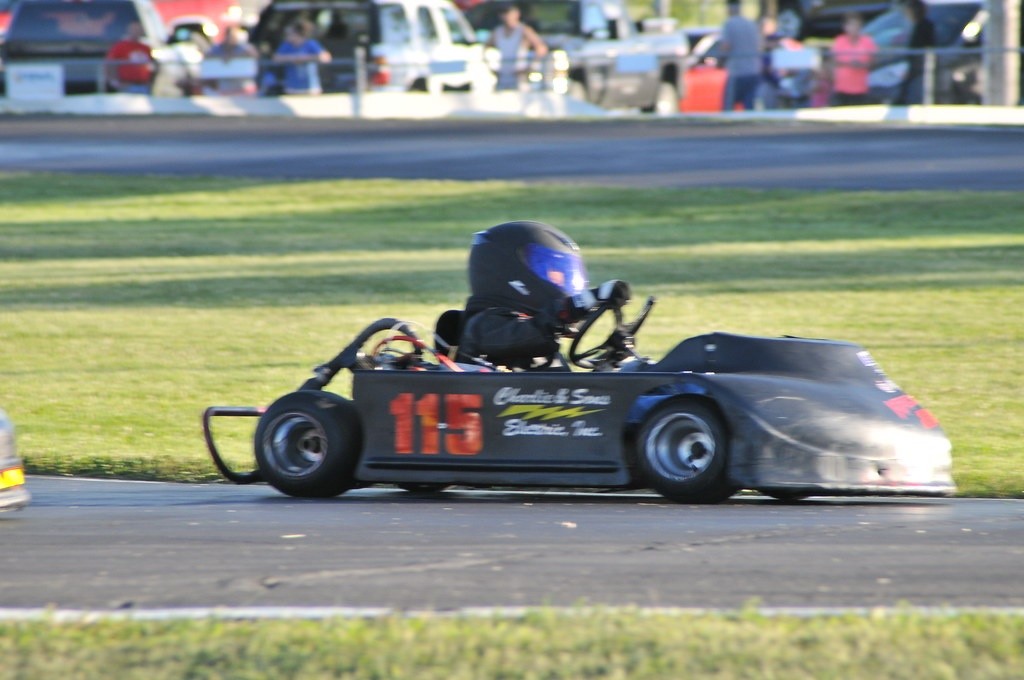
[[460, 0, 687, 119]]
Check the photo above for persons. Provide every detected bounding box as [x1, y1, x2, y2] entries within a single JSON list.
[[830, 14, 877, 105], [892, 1, 938, 105], [482, 7, 545, 90], [769, 27, 822, 107], [715, 0, 762, 111], [106, 21, 158, 96], [455, 220, 630, 372], [204, 24, 258, 94], [271, 17, 330, 96]]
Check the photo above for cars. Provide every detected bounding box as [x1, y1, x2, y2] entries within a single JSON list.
[[776, 0, 988, 106], [682, 31, 741, 111]]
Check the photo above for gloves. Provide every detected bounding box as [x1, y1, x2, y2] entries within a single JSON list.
[[563, 279, 631, 321]]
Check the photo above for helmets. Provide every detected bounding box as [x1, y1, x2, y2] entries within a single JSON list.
[[470, 220, 588, 317]]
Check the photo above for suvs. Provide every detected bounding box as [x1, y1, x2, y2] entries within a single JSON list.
[[247, 0, 498, 101], [1, 2, 168, 95]]
